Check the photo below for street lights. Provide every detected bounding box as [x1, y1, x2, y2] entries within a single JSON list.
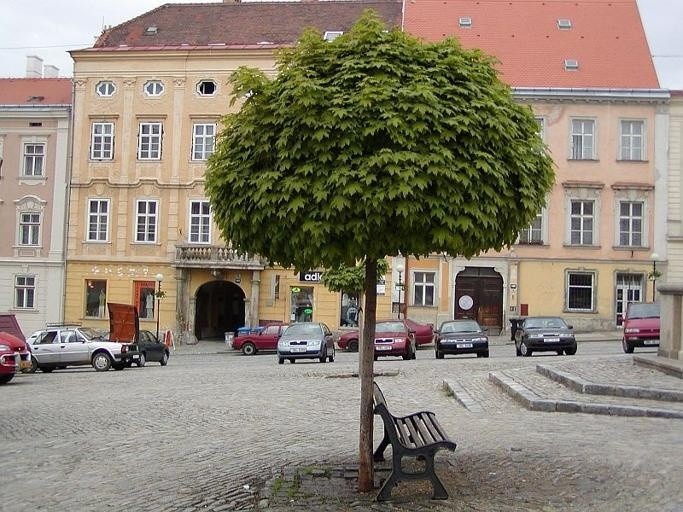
[[155, 272, 165, 337], [650, 252, 660, 302], [394, 263, 405, 318]]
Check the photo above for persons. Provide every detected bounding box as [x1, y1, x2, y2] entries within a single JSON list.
[[97, 287, 105, 317], [145, 291, 154, 318]]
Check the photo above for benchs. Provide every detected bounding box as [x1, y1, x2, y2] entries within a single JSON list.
[[368, 380, 458, 503]]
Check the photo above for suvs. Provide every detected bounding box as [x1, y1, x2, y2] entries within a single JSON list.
[[21, 320, 142, 373], [616, 299, 661, 353]]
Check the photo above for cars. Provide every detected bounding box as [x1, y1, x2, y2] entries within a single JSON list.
[[374, 318, 417, 360], [274, 320, 335, 365], [432, 317, 491, 360], [230, 320, 286, 356], [515, 316, 577, 356], [99, 324, 170, 371], [334, 315, 436, 352], [0, 327, 34, 386]]
